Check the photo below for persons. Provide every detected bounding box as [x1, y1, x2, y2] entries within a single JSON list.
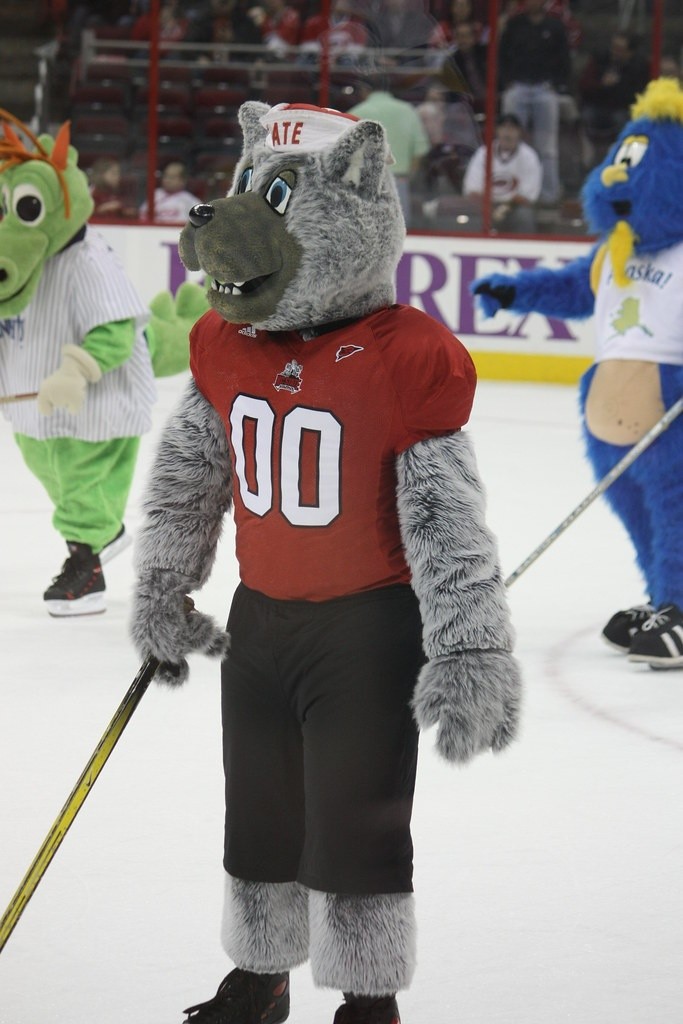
[[1, 121, 159, 615], [469, 75, 683, 668], [132, 98, 522, 1024], [90, 0, 683, 224]]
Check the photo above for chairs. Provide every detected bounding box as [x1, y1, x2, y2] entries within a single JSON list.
[[425, 195, 488, 233], [138, 104, 193, 139], [79, 79, 130, 108], [206, 137, 243, 173], [195, 170, 231, 201], [75, 103, 128, 137], [199, 80, 253, 105], [140, 134, 193, 174], [201, 100, 245, 137], [79, 134, 129, 168], [322, 76, 373, 114], [128, 74, 188, 108]]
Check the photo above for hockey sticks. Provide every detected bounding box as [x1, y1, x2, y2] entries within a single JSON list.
[[0, 392, 40, 405], [502, 397, 683, 588], [0, 595, 198, 951]]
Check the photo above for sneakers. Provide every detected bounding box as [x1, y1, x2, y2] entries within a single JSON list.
[[602, 604, 683, 653], [333, 998, 401, 1024], [182, 966, 290, 1024], [43, 543, 106, 617], [627, 603, 683, 670], [66, 523, 128, 567]]
